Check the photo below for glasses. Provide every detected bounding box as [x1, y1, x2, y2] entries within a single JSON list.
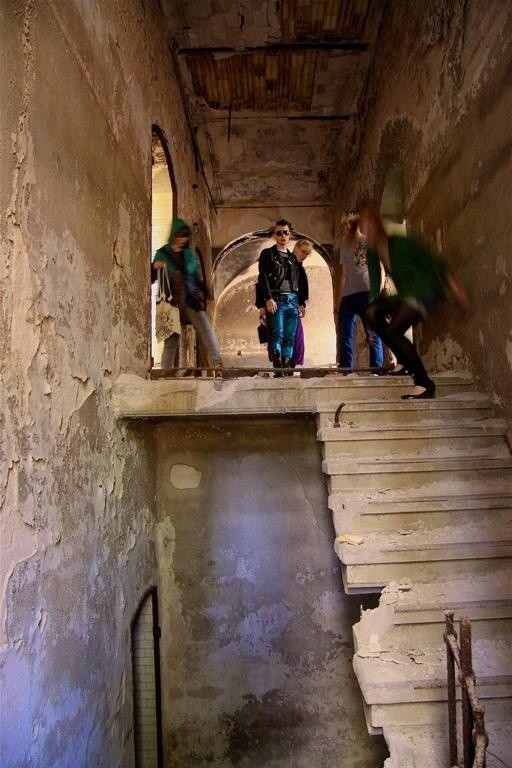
[[275, 229, 290, 237], [301, 249, 309, 256]]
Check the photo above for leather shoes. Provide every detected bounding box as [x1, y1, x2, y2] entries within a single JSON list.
[[401, 382, 436, 400], [387, 365, 411, 376]]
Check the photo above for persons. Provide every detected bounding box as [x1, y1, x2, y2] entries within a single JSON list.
[[256, 239, 313, 377], [259, 218, 300, 377], [334, 208, 385, 376], [151, 217, 223, 377], [358, 204, 455, 398]]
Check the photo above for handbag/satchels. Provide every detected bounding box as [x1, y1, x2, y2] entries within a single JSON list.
[[257, 324, 268, 343], [383, 276, 397, 298], [156, 295, 182, 340]]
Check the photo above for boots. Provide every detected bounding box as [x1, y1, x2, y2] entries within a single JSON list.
[[274, 356, 292, 377]]
